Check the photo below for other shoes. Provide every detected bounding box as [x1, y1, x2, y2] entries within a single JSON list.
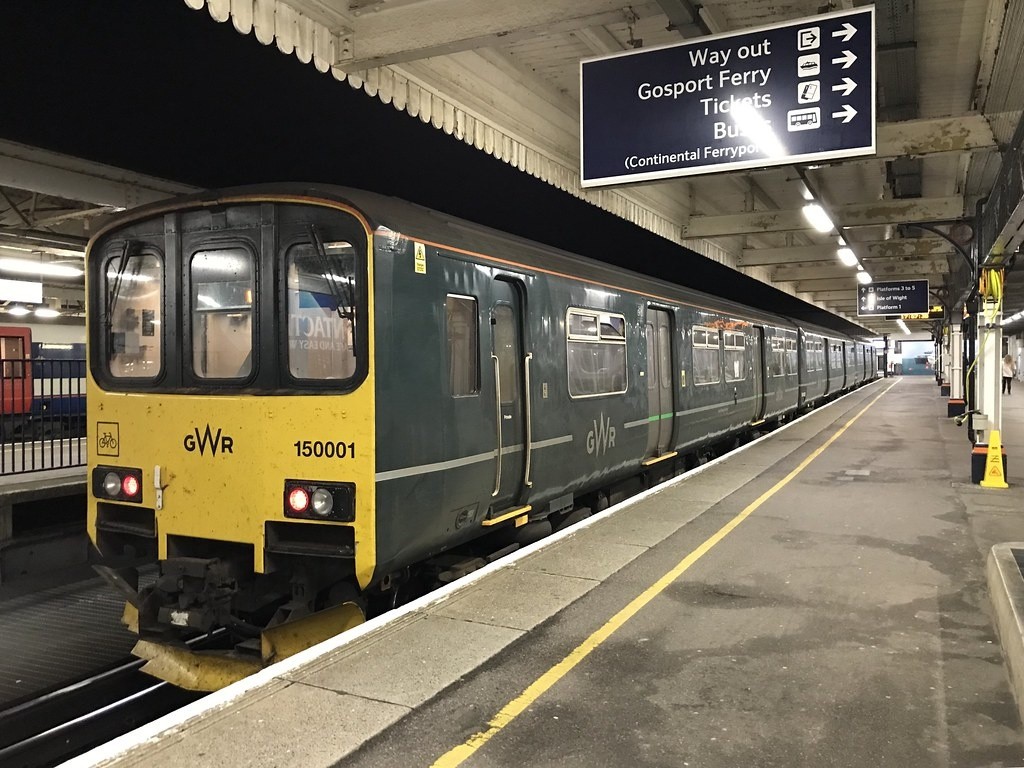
[[1008, 391, 1011, 395], [1002, 390, 1005, 393]]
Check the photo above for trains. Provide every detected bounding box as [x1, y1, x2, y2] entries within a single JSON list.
[[85, 194, 879, 664]]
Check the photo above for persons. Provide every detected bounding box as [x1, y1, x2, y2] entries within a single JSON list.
[[1002, 354, 1017, 395]]
